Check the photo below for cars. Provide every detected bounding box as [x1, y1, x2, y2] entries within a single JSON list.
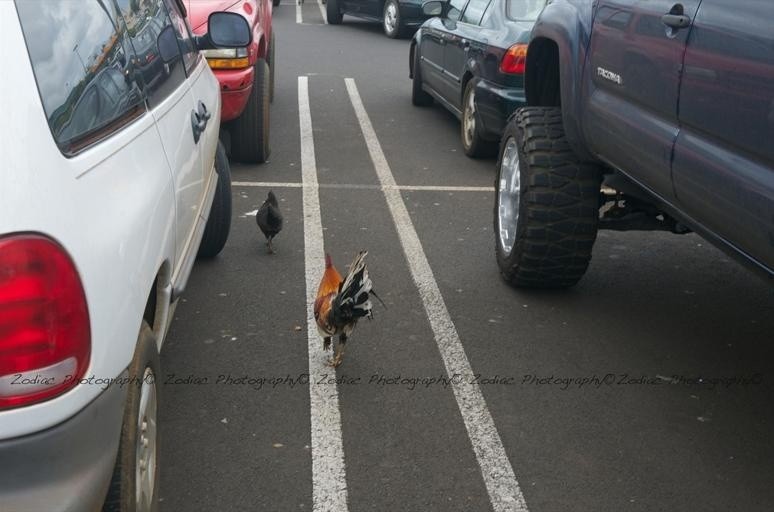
[[2, 0, 233, 511], [322, 0, 431, 39], [409, 0, 556, 158], [179, 0, 275, 166]]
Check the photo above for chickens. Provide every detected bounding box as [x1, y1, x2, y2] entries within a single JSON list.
[[314, 248, 388, 368], [256, 189, 283, 254]]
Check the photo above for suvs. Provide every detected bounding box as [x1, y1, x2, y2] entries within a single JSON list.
[[493, 0, 774, 292]]
